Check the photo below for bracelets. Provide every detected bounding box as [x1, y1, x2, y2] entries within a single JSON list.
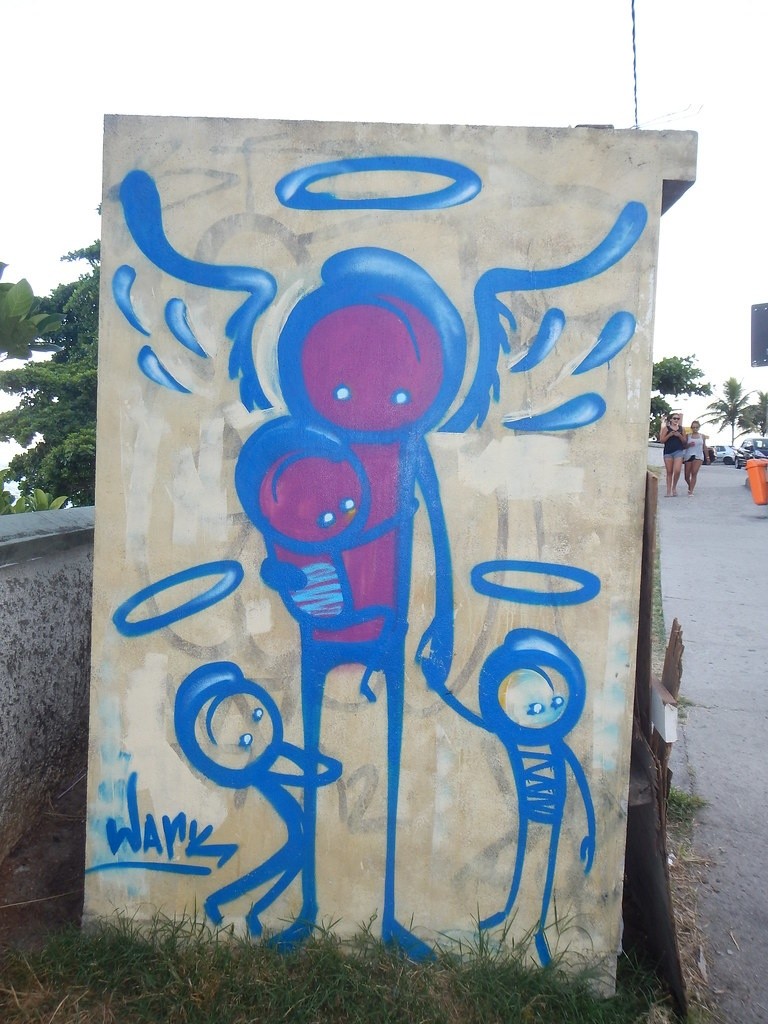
[[705, 457, 710, 459]]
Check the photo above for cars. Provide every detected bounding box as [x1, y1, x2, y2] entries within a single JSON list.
[[734, 437, 768, 469], [710, 444, 738, 465]]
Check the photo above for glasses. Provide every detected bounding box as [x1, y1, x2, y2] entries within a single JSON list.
[[672, 418, 680, 420], [693, 427, 699, 429]]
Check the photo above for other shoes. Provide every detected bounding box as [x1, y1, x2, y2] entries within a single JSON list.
[[673, 489, 677, 497], [688, 489, 694, 497], [664, 490, 671, 497]]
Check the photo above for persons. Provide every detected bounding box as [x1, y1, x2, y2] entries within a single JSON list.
[[683, 421, 711, 497], [660, 413, 687, 498]]
